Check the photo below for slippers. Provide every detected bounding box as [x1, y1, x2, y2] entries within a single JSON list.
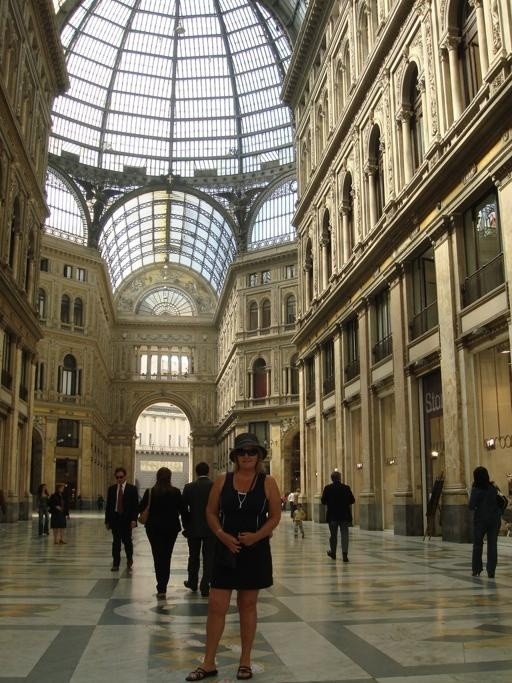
[[237, 664, 253, 680], [185, 666, 219, 681]]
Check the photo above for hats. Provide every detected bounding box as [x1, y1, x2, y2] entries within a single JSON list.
[[230, 432, 267, 462]]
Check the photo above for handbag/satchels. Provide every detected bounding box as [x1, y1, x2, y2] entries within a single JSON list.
[[495, 490, 509, 513], [137, 487, 154, 523]]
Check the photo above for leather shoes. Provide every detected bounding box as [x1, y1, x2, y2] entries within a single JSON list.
[[326, 549, 336, 559], [343, 559, 349, 562], [183, 580, 199, 591], [112, 563, 120, 571], [126, 558, 133, 567], [59, 539, 67, 544]]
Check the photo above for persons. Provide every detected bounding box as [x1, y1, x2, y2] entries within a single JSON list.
[[468, 465, 502, 578], [184, 433, 282, 681], [139, 468, 182, 599], [35, 484, 50, 537], [181, 462, 215, 597], [320, 471, 356, 562], [105, 466, 139, 572], [47, 483, 70, 545], [97, 493, 104, 512], [280, 488, 306, 539]]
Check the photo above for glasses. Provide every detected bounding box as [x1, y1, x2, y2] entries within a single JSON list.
[[237, 449, 259, 456], [115, 475, 124, 480]]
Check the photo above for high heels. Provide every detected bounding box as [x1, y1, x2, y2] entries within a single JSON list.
[[472, 568, 481, 576]]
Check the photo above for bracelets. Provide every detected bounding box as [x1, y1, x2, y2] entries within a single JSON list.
[[215, 528, 223, 536]]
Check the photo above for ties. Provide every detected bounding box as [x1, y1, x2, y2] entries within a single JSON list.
[[117, 484, 124, 514]]
